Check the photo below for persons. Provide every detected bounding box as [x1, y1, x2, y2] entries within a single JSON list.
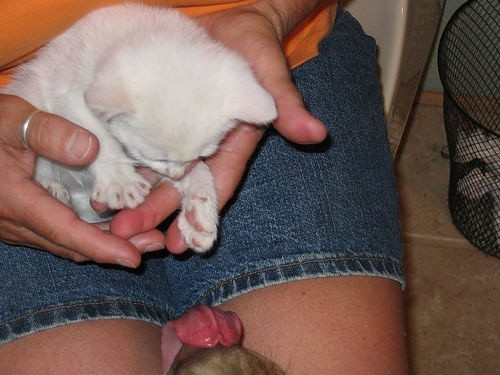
[[0, 0, 409, 375]]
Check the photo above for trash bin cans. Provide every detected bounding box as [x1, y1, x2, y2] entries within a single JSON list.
[[436, 0, 500, 259]]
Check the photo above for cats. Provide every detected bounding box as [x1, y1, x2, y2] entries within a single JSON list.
[[0, 3, 278, 255]]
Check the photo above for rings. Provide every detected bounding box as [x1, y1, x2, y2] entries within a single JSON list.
[[20, 109, 41, 148]]
